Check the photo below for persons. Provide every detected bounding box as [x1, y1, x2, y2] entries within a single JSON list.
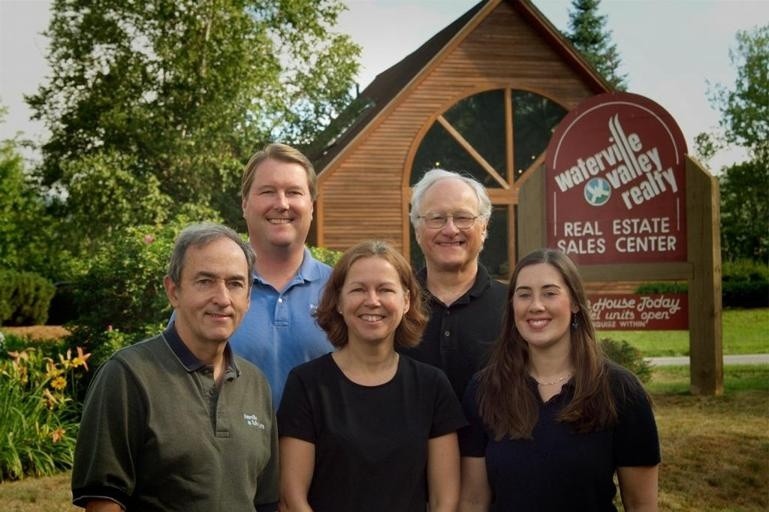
[[399, 168, 510, 386], [166, 141, 334, 411], [70, 224, 277, 505], [279, 240, 458, 512], [459, 249, 660, 512]]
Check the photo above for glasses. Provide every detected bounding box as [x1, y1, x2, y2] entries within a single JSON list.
[[415, 209, 484, 229]]
